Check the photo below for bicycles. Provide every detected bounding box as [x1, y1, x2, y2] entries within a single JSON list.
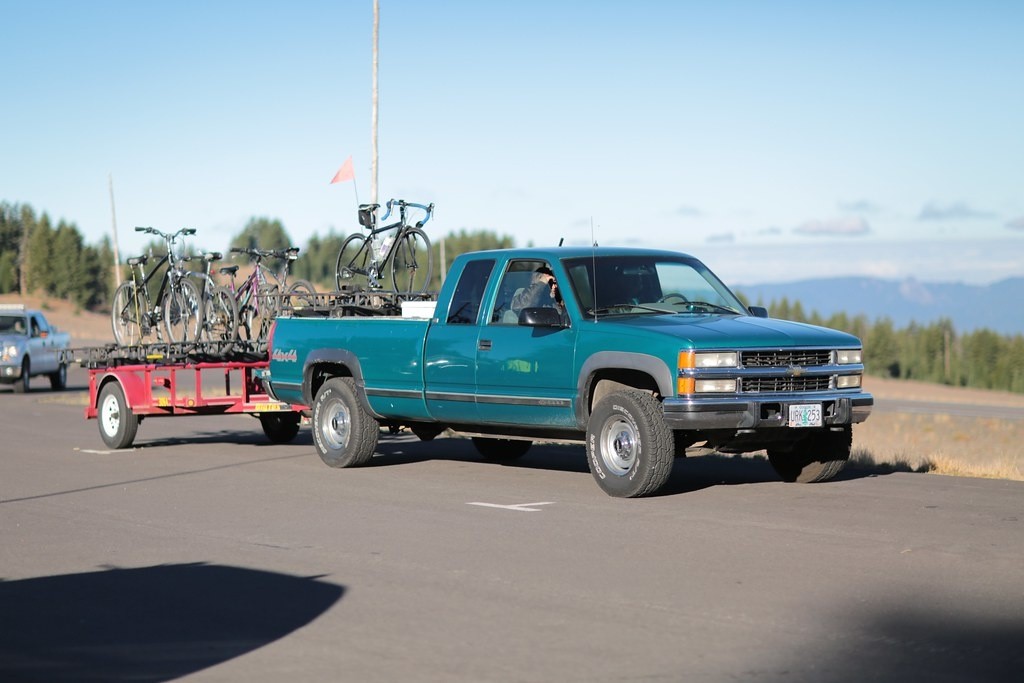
[[158, 246, 319, 353], [111, 224, 204, 354], [335, 201, 436, 295]]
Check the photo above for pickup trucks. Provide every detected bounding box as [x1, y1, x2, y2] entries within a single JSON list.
[[266, 244, 875, 498], [0, 303, 72, 395]]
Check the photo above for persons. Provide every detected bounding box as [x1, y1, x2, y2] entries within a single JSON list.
[[513, 267, 562, 321], [9, 320, 26, 334]]
